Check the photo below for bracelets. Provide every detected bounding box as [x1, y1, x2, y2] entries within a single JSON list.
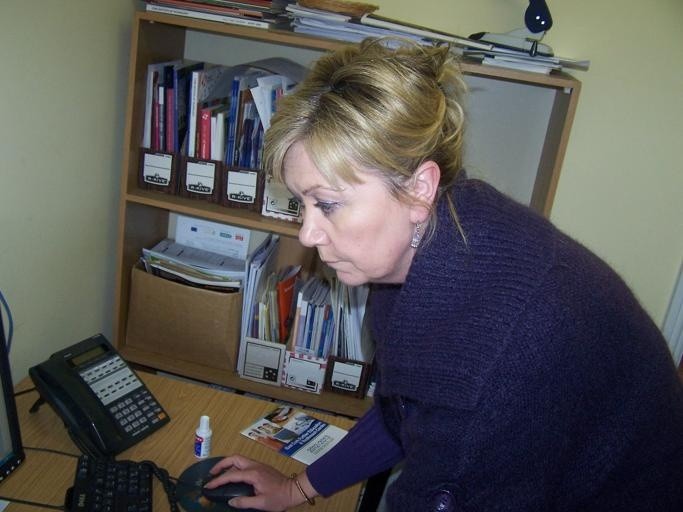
[[290, 473, 315, 506]]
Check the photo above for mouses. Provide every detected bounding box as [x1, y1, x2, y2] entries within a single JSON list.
[[201, 478, 255, 506]]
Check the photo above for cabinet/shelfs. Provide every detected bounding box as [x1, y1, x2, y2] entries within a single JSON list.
[[112, 7, 584, 422]]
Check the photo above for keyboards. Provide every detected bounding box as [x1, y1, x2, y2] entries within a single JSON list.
[[64, 453, 154, 512]]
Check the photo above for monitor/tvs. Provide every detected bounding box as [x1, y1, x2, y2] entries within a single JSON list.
[[0, 306, 27, 487]]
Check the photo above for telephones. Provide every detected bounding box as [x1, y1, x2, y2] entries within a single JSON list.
[[28, 332, 170, 462]]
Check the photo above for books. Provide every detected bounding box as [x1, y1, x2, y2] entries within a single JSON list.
[[142, 0, 565, 220], [142, 213, 374, 364], [240, 404, 350, 465]]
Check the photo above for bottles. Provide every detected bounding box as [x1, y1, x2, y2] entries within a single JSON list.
[[193, 415, 213, 460]]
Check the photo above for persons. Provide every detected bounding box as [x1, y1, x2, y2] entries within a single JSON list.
[[203, 38, 683, 512]]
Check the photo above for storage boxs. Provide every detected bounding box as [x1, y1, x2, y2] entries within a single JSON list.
[[122, 260, 244, 374]]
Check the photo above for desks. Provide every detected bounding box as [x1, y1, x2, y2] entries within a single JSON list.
[[1, 372, 368, 510]]
